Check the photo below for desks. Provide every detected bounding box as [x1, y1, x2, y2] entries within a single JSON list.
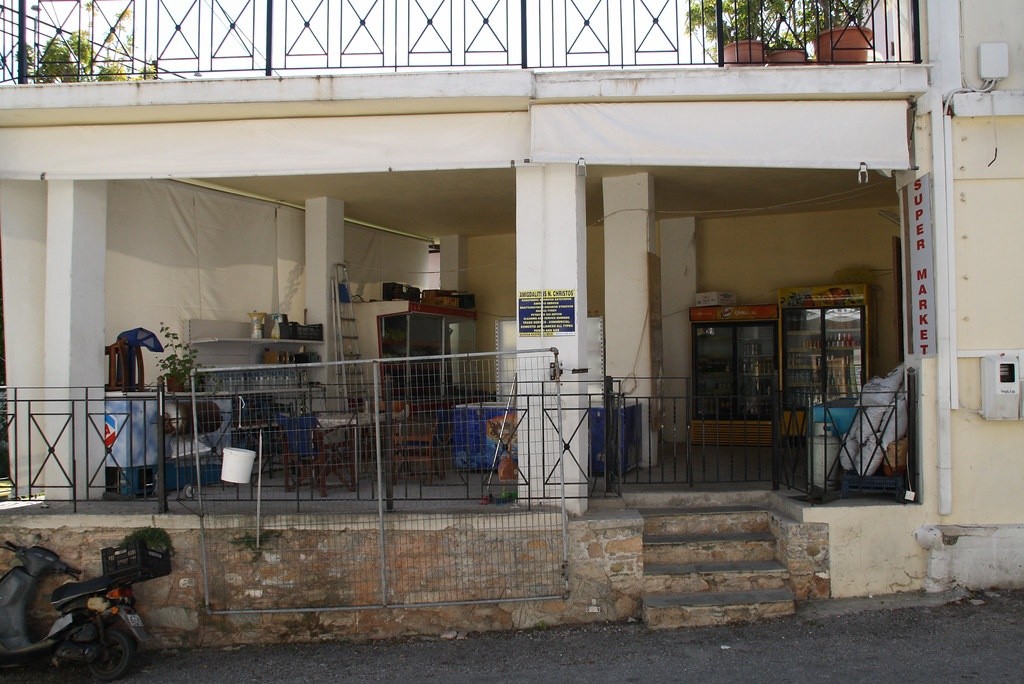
[[316, 412, 404, 491]]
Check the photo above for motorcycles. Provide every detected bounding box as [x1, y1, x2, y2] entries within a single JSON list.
[[0, 538, 174, 684]]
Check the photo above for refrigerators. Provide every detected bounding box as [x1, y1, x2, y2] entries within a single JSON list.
[[689, 304, 778, 444], [777, 284, 878, 436]]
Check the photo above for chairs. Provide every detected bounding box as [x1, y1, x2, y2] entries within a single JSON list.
[[278, 416, 355, 497], [392, 418, 438, 486]]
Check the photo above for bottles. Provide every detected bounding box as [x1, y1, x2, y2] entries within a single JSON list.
[[790, 370, 810, 384], [826, 350, 854, 364], [205, 370, 297, 391], [827, 331, 852, 347], [742, 402, 764, 420], [800, 336, 820, 348], [790, 350, 821, 365], [830, 367, 852, 385], [796, 388, 811, 408], [744, 358, 773, 373], [705, 394, 730, 414]]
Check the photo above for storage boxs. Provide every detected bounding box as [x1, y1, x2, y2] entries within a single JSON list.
[[696, 291, 738, 308], [101, 540, 172, 583]]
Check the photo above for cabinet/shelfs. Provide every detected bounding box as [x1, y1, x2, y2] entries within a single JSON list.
[[189, 319, 326, 475]]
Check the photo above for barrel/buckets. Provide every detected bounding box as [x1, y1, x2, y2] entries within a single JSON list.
[[220, 446, 257, 484], [288, 417, 317, 457]]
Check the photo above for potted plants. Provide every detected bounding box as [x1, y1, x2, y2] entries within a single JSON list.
[[809, 0, 874, 65], [763, 0, 809, 66], [685, 0, 766, 67], [155, 320, 201, 392]]
